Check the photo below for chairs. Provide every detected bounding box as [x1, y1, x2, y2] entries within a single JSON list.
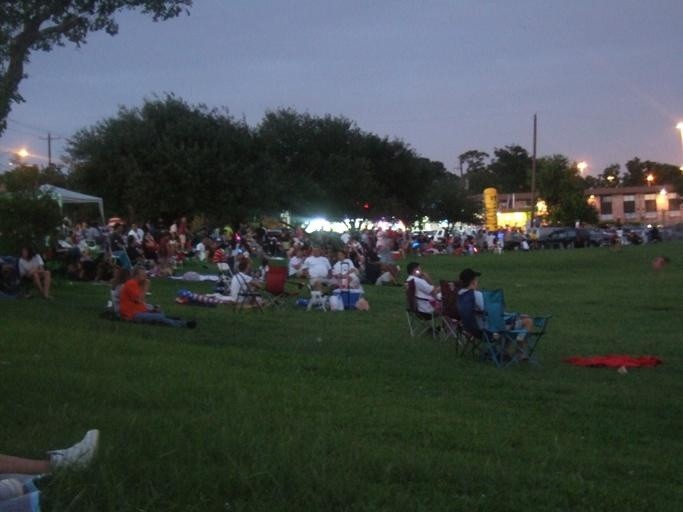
[[404, 278, 548, 369], [305, 284, 326, 312], [233, 272, 265, 315]]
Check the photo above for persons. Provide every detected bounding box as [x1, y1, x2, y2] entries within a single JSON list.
[[611, 219, 661, 247], [405, 262, 531, 358], [2, 243, 52, 299], [1, 429, 99, 474], [360, 223, 537, 254], [59, 217, 404, 328]]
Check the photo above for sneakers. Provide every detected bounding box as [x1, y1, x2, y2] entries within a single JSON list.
[[46, 428, 99, 479]]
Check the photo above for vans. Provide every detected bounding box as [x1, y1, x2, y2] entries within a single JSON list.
[[269, 227, 310, 240]]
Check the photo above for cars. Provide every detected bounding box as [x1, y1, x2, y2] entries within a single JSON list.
[[416, 222, 682, 248]]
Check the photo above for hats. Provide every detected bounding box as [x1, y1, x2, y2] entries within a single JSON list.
[[460, 269, 482, 280]]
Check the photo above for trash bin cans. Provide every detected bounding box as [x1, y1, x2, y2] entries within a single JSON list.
[[264, 267, 288, 294]]
